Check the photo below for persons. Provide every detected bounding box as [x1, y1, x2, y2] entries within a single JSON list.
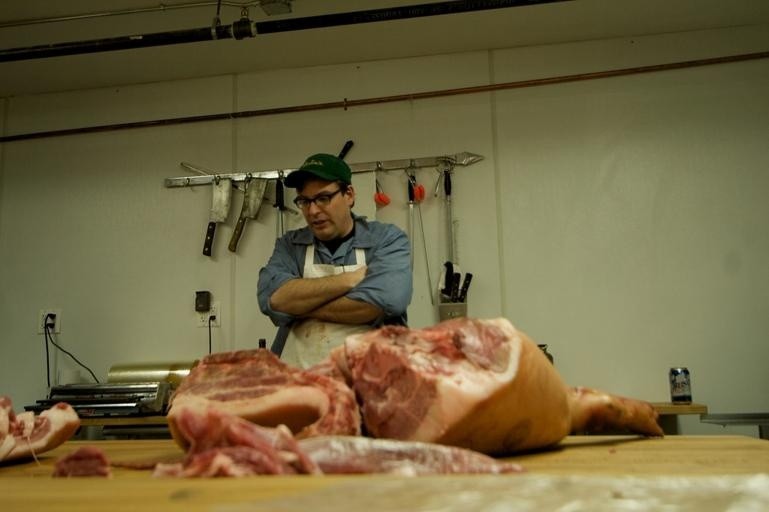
[[256, 154, 413, 370]]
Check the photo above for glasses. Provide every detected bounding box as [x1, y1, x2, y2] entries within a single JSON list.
[[293, 188, 341, 207]]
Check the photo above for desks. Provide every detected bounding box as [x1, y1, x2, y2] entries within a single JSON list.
[[648, 399, 707, 434], [73, 415, 169, 439], [698, 412, 768, 439], [1, 433, 769, 511]]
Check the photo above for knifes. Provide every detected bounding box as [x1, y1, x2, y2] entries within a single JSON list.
[[441, 261, 473, 303], [228, 177, 268, 251], [202, 177, 232, 256]]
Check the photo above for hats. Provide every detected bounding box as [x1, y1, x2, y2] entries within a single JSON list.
[[283, 153, 351, 187]]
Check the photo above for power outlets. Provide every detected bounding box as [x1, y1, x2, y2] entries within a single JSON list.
[[37, 308, 60, 335], [195, 302, 222, 328]]
